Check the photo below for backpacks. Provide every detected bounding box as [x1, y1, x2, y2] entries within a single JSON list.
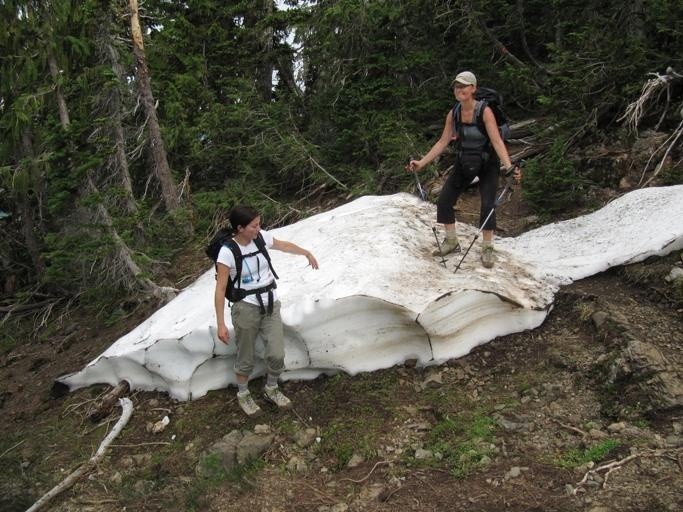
[[452, 99, 508, 143], [204, 225, 277, 302]]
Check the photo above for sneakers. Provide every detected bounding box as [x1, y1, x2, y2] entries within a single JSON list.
[[263, 383, 292, 410], [236, 389, 264, 419], [479, 242, 495, 268], [432, 236, 461, 257]]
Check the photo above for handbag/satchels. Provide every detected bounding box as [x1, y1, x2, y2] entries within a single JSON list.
[[452, 147, 491, 186]]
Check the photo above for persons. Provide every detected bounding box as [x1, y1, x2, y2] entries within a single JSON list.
[[213, 204, 319, 419], [405, 71, 522, 269]]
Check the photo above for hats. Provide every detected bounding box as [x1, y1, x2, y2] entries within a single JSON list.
[[451, 70, 477, 86]]
